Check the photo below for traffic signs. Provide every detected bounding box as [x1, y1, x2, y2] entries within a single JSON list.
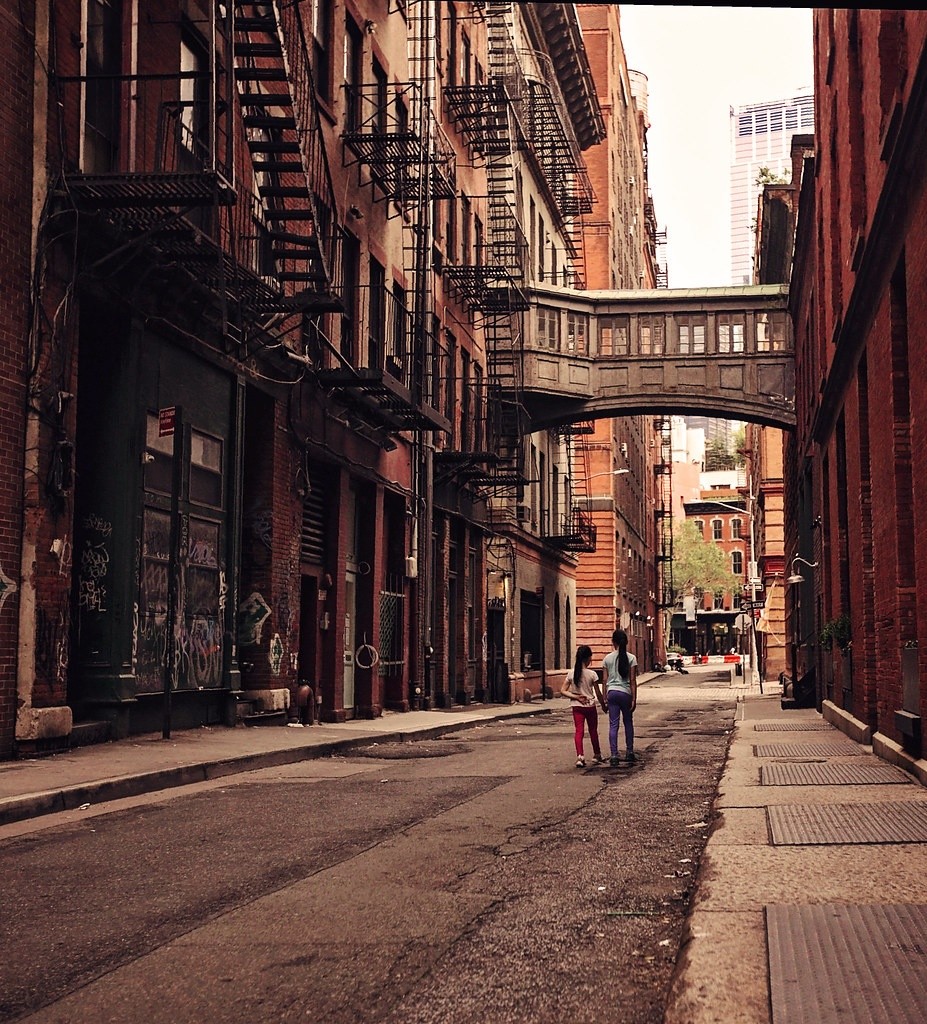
[[740, 601, 764, 612], [743, 583, 763, 592]]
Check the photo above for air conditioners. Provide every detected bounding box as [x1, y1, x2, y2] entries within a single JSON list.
[[640, 270, 645, 279], [629, 176, 635, 186], [621, 443, 627, 452], [518, 505, 530, 523], [629, 226, 633, 236]]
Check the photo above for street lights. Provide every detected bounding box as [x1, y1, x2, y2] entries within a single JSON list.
[[691, 500, 759, 685]]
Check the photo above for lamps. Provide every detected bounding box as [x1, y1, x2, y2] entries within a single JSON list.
[[365, 19, 377, 34], [350, 204, 364, 219], [786, 558, 818, 584]]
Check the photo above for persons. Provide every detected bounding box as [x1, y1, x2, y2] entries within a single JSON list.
[[729, 646, 737, 655], [560, 645, 609, 767], [602, 629, 638, 766]]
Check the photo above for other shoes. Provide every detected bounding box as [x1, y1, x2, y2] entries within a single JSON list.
[[625, 752, 639, 762], [592, 753, 606, 764], [609, 756, 619, 766], [576, 755, 587, 768]]
